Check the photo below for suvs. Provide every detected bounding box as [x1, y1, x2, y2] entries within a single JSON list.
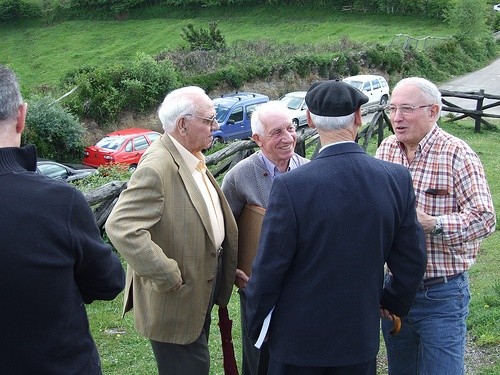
[[342, 73, 390, 116]]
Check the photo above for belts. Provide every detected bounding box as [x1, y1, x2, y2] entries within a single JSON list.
[[386, 273, 463, 286]]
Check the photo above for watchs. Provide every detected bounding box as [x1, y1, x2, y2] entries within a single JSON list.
[[432, 215, 444, 237]]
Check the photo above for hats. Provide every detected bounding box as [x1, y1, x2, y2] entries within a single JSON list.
[[305, 79, 369, 117]]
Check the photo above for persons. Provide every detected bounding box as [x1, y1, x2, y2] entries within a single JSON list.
[[219, 101, 313, 375], [105, 86, 241, 375], [1, 66, 126, 375], [246, 80, 428, 375], [370, 78, 498, 375]]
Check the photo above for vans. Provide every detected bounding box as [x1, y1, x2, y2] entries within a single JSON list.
[[207, 91, 269, 148]]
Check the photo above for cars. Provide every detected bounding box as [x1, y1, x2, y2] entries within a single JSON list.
[[278, 91, 309, 130], [33, 156, 98, 185], [83, 126, 163, 172]]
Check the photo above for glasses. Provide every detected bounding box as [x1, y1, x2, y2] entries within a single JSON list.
[[184, 113, 218, 126], [384, 103, 433, 115]]
